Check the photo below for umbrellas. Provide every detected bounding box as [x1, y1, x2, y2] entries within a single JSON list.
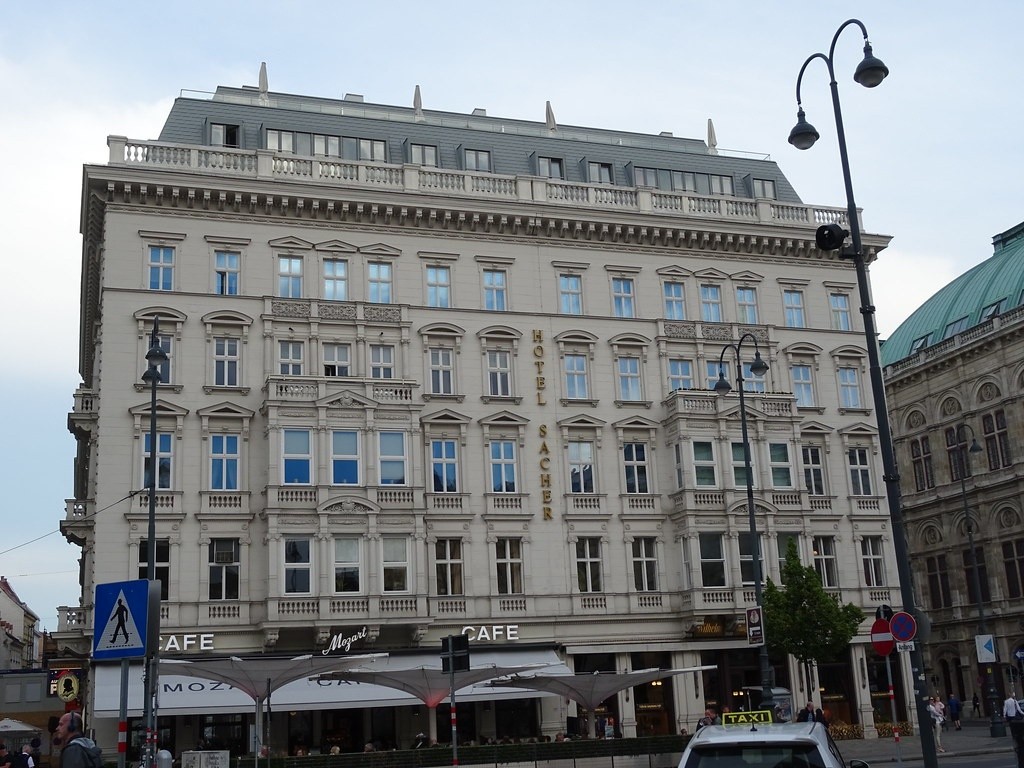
[[0, 717, 42, 751]]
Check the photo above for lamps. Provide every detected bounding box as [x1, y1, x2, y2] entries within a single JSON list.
[[652, 680, 662, 686]]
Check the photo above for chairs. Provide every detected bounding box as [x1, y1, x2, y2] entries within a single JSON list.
[[771, 753, 808, 768], [709, 749, 748, 768]]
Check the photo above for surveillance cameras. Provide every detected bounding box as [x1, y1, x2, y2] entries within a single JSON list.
[[816, 224, 849, 250]]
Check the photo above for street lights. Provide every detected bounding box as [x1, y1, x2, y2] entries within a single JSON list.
[[140, 315, 168, 768], [787, 16, 939, 768], [712, 331, 779, 722]]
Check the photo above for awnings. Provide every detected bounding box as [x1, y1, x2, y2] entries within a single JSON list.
[[93, 646, 580, 719]]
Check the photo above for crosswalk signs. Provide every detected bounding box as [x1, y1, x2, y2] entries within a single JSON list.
[[92, 579, 148, 660]]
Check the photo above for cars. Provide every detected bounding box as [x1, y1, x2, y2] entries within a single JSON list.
[[677, 722, 871, 768]]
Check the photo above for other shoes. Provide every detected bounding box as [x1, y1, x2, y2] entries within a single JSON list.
[[937, 748, 945, 752]]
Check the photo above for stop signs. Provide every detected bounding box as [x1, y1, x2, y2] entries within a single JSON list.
[[871, 618, 895, 654]]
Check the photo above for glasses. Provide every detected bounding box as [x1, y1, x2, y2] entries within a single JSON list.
[[930, 699, 933, 701]]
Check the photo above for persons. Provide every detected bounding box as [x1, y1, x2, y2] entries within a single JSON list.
[[0, 693, 1024, 768]]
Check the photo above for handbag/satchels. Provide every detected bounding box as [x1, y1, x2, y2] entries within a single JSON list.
[[931, 718, 936, 728]]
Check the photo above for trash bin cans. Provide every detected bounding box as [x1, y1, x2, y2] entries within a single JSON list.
[[156, 750, 172, 768]]
